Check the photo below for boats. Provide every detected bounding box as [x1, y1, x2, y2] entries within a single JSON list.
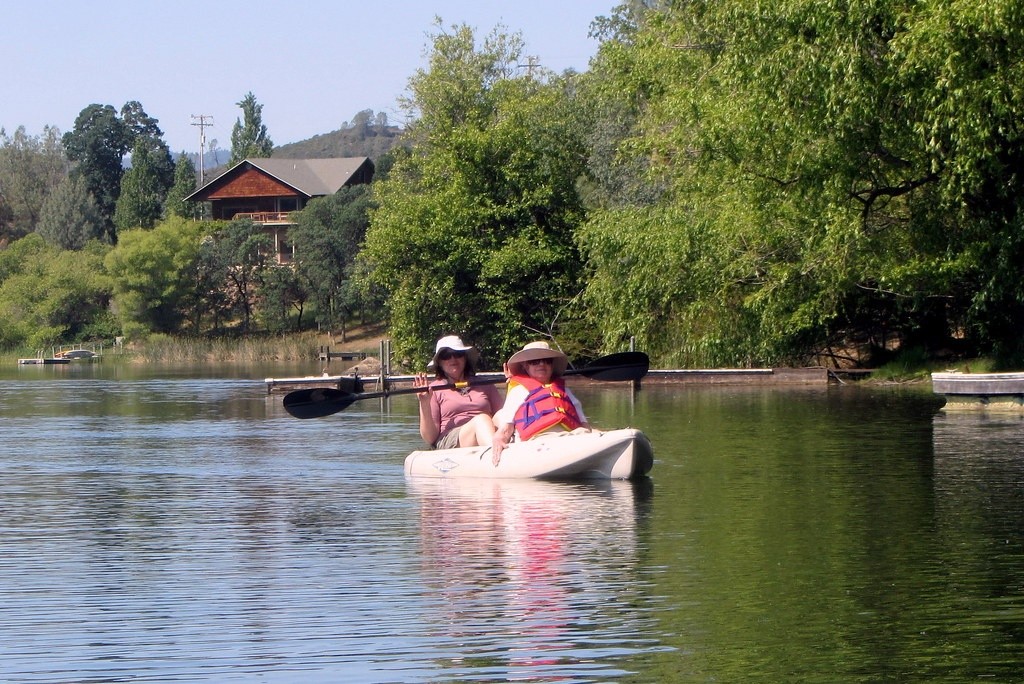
[[403, 427, 655, 483]]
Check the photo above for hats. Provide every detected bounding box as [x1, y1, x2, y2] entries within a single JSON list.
[[428, 336, 479, 371], [507, 341, 569, 379]]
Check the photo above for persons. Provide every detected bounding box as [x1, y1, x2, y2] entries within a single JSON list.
[[412, 335, 505, 450], [491, 341, 602, 468]]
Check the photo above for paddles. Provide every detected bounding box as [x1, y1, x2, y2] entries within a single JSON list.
[[282, 351, 650, 420]]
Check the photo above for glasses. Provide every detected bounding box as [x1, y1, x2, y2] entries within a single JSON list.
[[439, 350, 465, 360], [526, 358, 553, 365]]
